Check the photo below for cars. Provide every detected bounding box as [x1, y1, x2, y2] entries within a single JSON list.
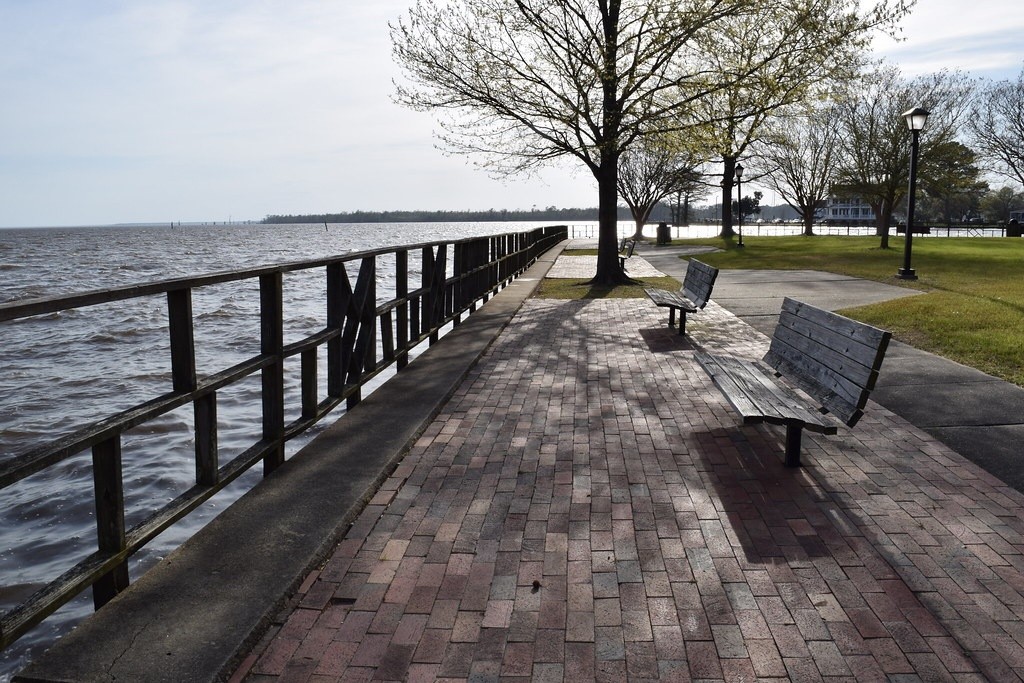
[[754, 217, 805, 224]]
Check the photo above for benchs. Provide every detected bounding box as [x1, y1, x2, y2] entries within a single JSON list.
[[618, 239, 636, 268], [692, 296, 892, 468], [896, 225, 931, 238], [644, 257, 719, 336], [618, 238, 627, 253]]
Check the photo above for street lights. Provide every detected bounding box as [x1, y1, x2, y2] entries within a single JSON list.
[[895, 106, 931, 280], [734, 163, 746, 249]]
